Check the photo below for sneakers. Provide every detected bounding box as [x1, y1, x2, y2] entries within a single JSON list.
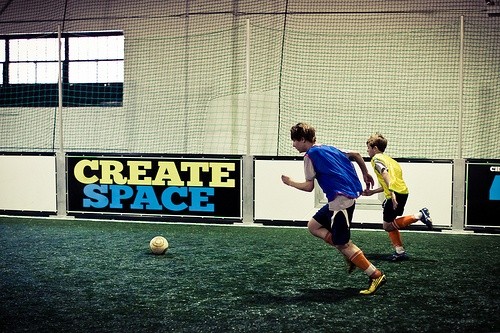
[[387, 251, 407, 261], [348, 262, 357, 273], [360, 272, 387, 294], [419, 207, 432, 229]]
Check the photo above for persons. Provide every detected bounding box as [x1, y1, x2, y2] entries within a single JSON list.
[[281, 122, 387, 295], [362, 133, 432, 262]]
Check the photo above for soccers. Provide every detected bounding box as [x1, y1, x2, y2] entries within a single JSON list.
[[149, 236, 168, 254]]
